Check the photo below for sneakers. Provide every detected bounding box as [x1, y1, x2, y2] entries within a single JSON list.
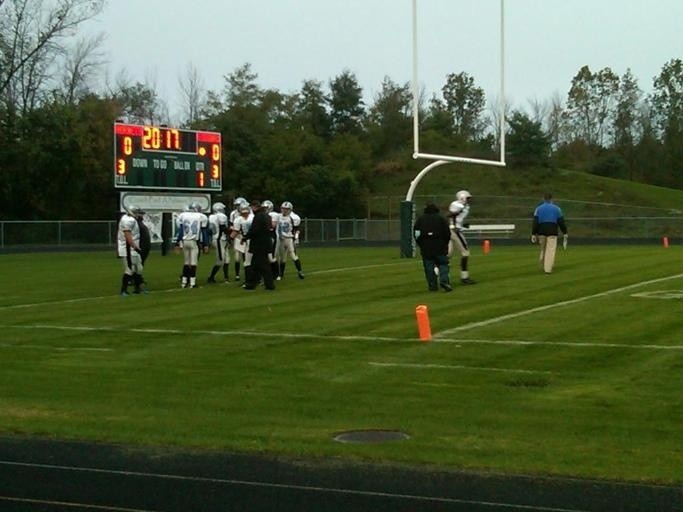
[[276, 276, 282, 280], [427, 278, 476, 292], [179, 275, 240, 289], [298, 270, 305, 279]]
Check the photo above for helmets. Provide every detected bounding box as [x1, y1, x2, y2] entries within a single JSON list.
[[455, 190, 472, 204], [127, 204, 139, 216], [187, 201, 202, 211], [280, 201, 293, 216], [261, 200, 274, 213], [213, 196, 250, 216]]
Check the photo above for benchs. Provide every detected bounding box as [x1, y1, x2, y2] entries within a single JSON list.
[[462, 223, 516, 238]]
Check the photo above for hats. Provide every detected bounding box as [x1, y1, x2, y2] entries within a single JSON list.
[[249, 200, 260, 207], [138, 209, 145, 214]]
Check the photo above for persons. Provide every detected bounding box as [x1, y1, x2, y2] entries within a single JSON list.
[[175, 196, 305, 291], [434, 190, 477, 284], [117, 204, 150, 297], [128, 210, 151, 286], [531, 193, 568, 273], [413, 200, 453, 292]]
